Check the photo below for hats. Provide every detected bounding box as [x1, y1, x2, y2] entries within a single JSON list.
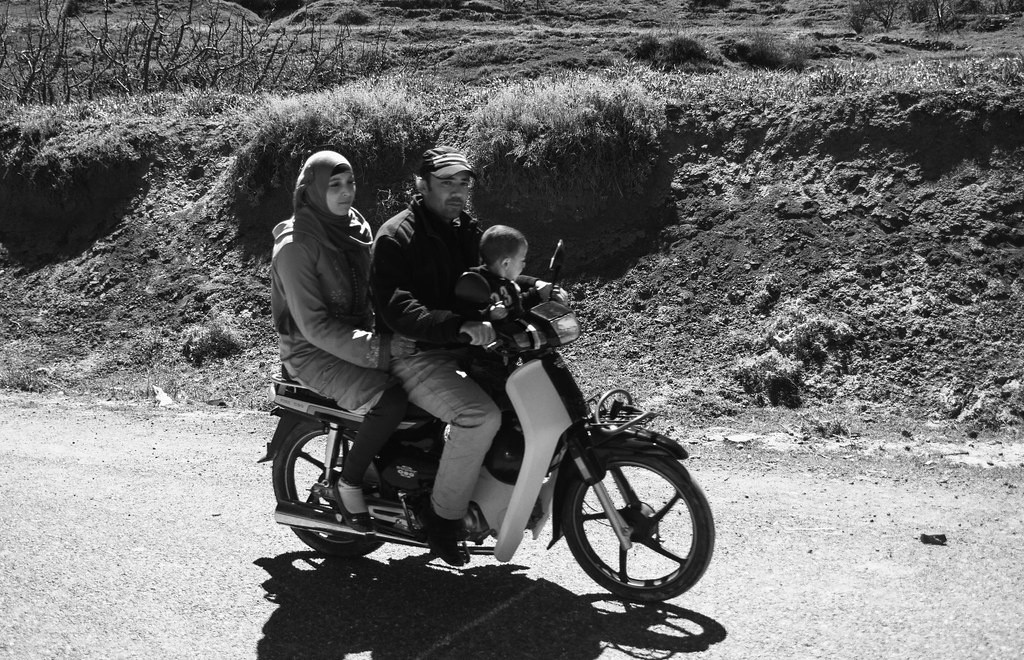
[[418, 147, 477, 182]]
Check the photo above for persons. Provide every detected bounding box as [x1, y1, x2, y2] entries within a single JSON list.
[[266, 145, 573, 565]]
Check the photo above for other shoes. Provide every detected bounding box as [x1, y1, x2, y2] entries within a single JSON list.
[[428, 517, 470, 566], [332, 479, 372, 532]]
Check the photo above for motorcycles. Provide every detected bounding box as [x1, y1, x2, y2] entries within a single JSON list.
[[256, 237, 716, 606]]
